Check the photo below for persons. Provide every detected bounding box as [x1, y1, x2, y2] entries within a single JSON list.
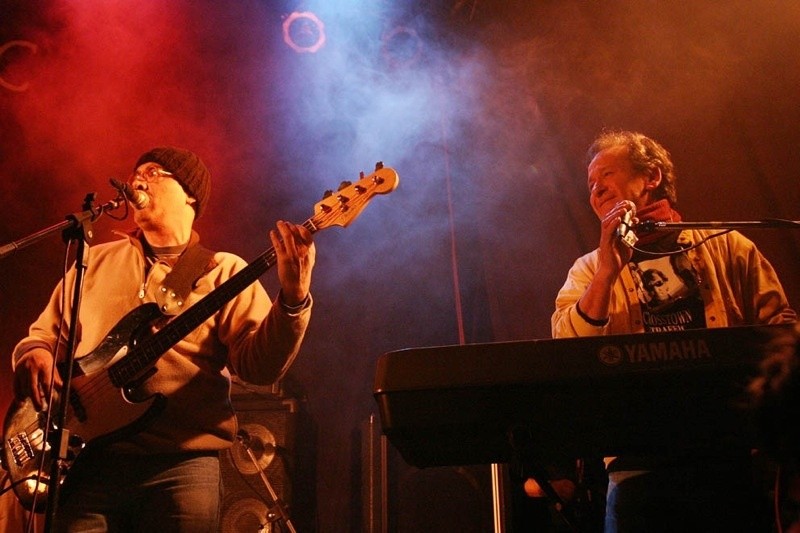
[[549, 131, 800, 533], [11, 147, 316, 533]]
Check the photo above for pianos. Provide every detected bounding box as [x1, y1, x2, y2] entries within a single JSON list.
[[372, 322, 800, 533]]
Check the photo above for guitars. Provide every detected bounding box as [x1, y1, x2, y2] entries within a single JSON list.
[[2, 161, 401, 513]]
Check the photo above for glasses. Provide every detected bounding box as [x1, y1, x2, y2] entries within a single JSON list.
[[126, 167, 173, 187]]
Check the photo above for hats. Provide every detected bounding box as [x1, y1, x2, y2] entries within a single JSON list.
[[135, 146, 210, 220]]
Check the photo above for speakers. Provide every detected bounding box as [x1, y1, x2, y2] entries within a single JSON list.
[[217, 397, 297, 533]]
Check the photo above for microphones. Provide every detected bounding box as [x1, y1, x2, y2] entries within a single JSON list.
[[616, 199, 637, 238], [109, 176, 150, 210]]
